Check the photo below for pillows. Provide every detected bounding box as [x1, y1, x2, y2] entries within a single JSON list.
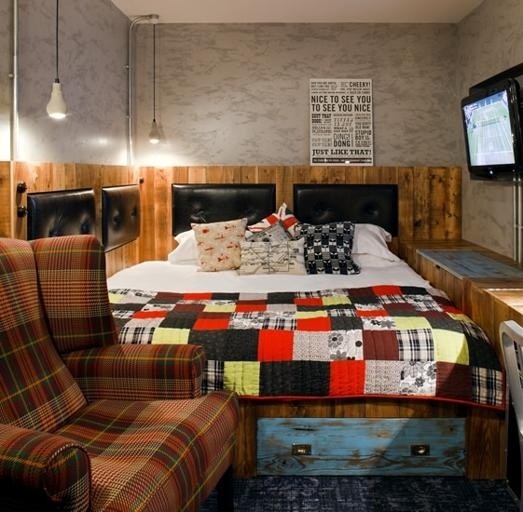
[[237, 236, 308, 275], [247, 220, 295, 242], [189, 216, 248, 271], [168, 227, 253, 265], [301, 221, 361, 275], [293, 223, 401, 261], [246, 202, 303, 240]]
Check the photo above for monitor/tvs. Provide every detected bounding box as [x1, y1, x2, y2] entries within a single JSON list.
[[461, 78, 523, 179]]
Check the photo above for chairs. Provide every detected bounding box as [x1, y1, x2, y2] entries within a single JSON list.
[[0, 235, 240, 512], [499, 319, 523, 471]]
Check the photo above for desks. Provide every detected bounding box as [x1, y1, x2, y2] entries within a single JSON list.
[[399, 240, 522, 366]]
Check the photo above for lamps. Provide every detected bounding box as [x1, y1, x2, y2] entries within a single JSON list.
[[149, 26, 162, 146], [46, 1, 69, 119]]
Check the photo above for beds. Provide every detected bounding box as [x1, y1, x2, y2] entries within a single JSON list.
[[105, 164, 507, 479]]
